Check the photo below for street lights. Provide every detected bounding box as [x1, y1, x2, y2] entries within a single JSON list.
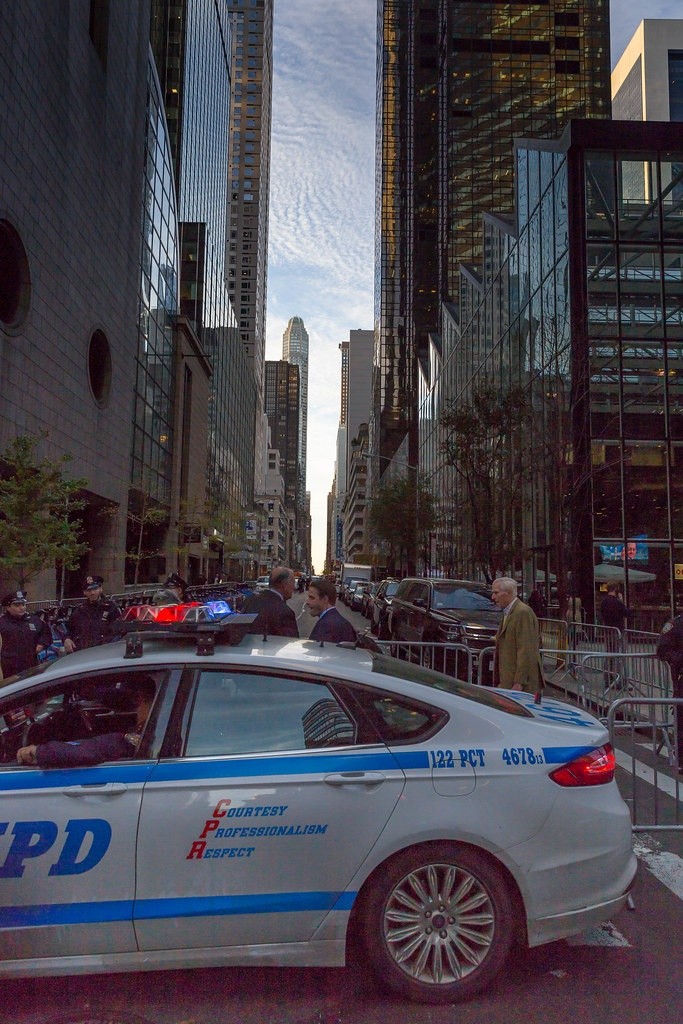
[[359, 452, 422, 577], [252, 496, 277, 585]]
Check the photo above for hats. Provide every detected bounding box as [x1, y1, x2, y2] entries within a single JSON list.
[[1, 590, 29, 605], [163, 572, 189, 590], [82, 576, 105, 590]]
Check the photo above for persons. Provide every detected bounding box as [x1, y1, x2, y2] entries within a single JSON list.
[[305, 578, 358, 645], [236, 566, 302, 638], [159, 573, 191, 609], [296, 574, 341, 593], [15, 674, 160, 767], [490, 577, 546, 700], [192, 570, 232, 590], [526, 590, 545, 635], [602, 538, 645, 569], [656, 607, 683, 776], [599, 580, 634, 692], [62, 576, 126, 656], [0, 590, 52, 680], [562, 589, 582, 646]]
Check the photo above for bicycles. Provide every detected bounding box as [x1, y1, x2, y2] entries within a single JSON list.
[[30, 583, 254, 668]]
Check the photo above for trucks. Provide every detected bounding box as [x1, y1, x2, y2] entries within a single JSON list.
[[338, 563, 372, 603], [331, 570, 340, 583]]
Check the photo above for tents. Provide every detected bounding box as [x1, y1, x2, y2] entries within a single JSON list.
[[567, 561, 656, 584], [504, 568, 556, 583]]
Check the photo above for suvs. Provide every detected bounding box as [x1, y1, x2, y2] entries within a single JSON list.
[[390, 578, 517, 671], [341, 576, 369, 600], [372, 579, 406, 641]]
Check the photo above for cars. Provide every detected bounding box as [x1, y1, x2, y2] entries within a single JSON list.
[[256, 575, 272, 591], [351, 582, 373, 609], [296, 573, 334, 594], [360, 582, 383, 616], [345, 580, 370, 604], [333, 577, 340, 596], [1, 600, 641, 1003]]
